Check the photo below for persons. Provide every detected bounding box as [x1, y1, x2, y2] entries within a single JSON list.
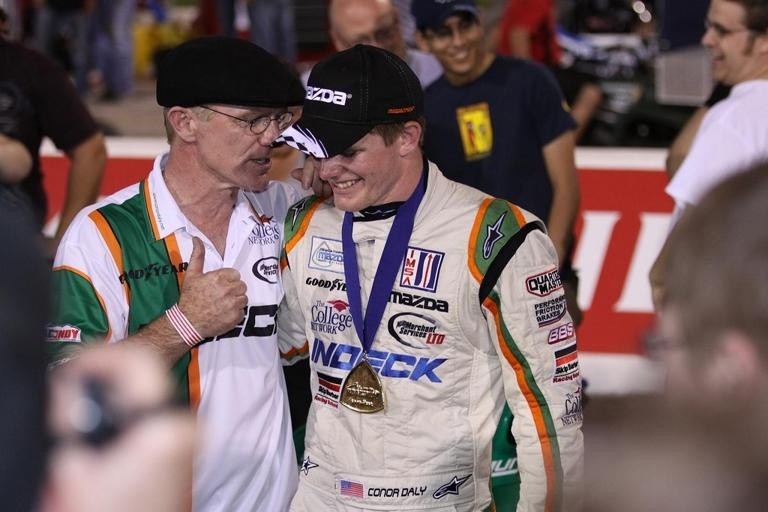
[[1, 0, 768, 511]]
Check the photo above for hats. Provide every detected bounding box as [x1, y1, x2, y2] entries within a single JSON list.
[[410, 0, 481, 31], [268, 42, 426, 161], [155, 36, 307, 108]]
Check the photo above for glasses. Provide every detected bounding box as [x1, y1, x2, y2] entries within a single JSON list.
[[704, 18, 757, 38], [199, 104, 296, 135], [646, 329, 715, 359]]
